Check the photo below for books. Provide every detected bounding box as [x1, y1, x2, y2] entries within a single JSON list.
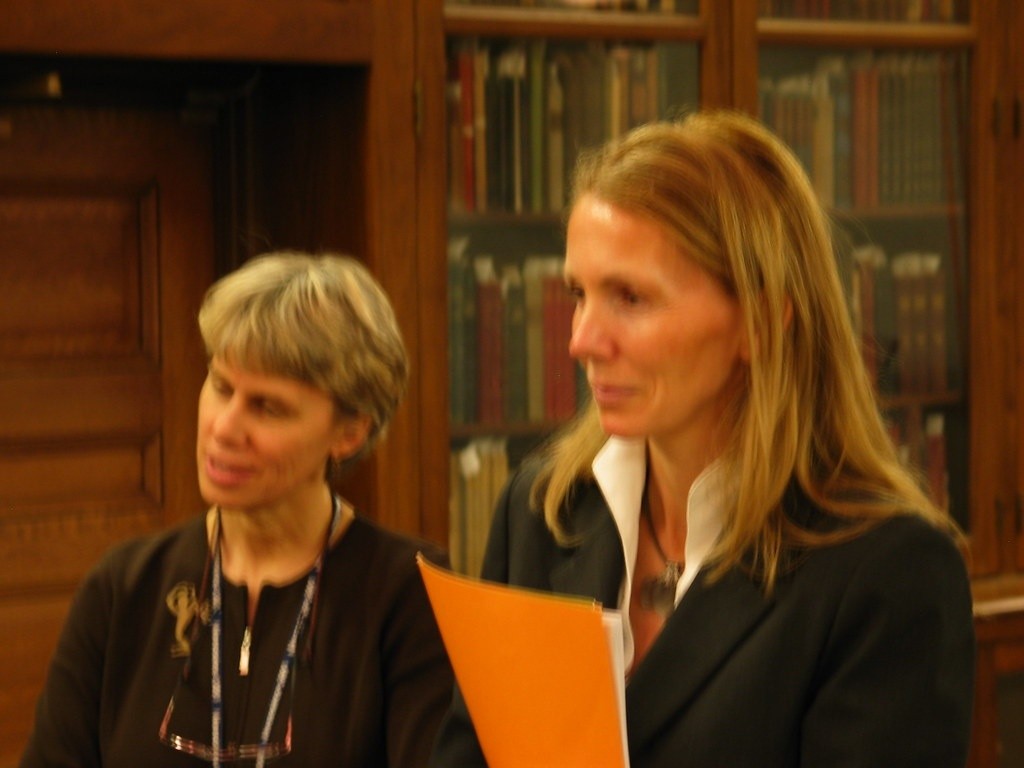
[[446, 0, 954, 577]]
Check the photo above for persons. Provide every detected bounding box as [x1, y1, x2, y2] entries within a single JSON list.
[[16, 251, 489, 768], [482, 110, 973, 768]]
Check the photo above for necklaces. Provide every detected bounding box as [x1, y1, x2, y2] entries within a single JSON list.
[[637, 484, 684, 613]]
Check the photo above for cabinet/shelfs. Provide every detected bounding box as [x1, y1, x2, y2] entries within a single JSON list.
[[414, 1, 1023, 640]]
